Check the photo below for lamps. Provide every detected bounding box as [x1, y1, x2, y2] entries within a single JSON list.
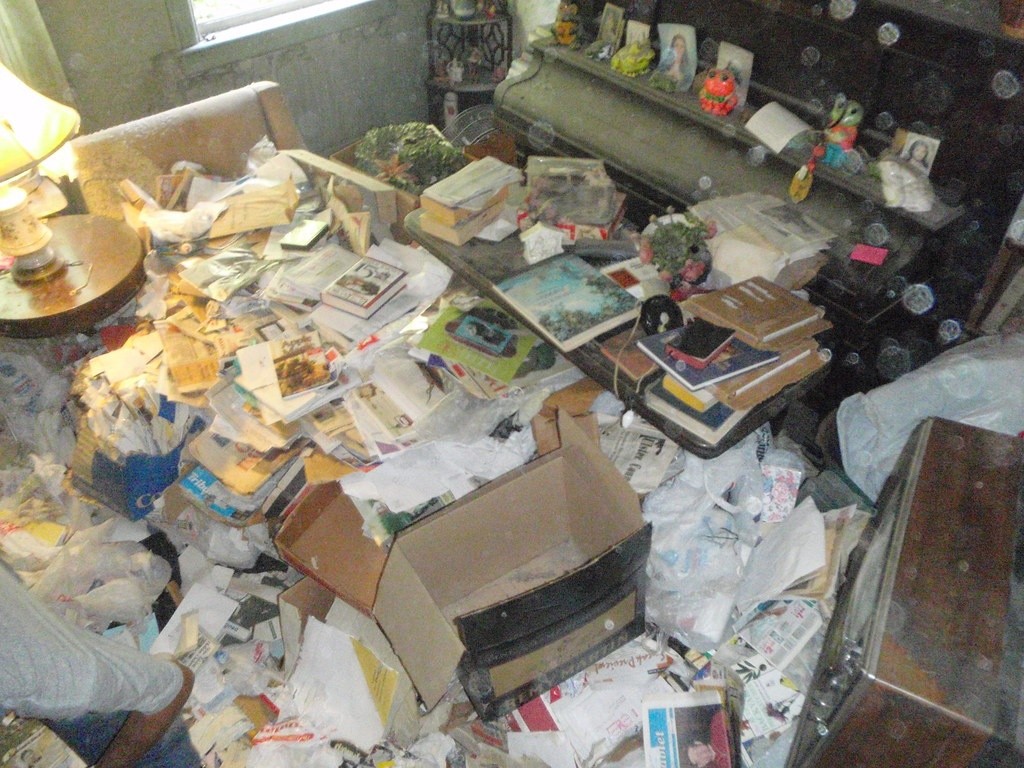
[[0, 61, 80, 287]]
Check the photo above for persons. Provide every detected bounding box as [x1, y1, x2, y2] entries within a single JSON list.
[[660, 34, 692, 84], [685, 710, 731, 768], [1, 567, 196, 768], [602, 12, 617, 40], [908, 142, 930, 171]]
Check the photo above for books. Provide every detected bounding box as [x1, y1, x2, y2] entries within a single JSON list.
[[494, 251, 643, 350], [176, 439, 313, 526], [418, 160, 520, 246], [322, 256, 406, 319], [600, 276, 822, 445]]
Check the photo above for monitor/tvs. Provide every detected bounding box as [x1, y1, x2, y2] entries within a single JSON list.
[[784, 417, 1024, 768]]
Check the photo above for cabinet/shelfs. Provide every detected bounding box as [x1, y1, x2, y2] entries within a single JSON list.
[[426, 1, 515, 135]]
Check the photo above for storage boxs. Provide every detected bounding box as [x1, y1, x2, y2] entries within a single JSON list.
[[68, 400, 202, 521], [274, 378, 653, 763], [329, 125, 476, 230], [517, 183, 627, 245]]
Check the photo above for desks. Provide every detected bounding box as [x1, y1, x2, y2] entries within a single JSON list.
[[403, 176, 836, 459], [0, 213, 147, 341]]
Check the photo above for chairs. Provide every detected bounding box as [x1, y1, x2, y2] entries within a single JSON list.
[[57, 78, 397, 271]]
